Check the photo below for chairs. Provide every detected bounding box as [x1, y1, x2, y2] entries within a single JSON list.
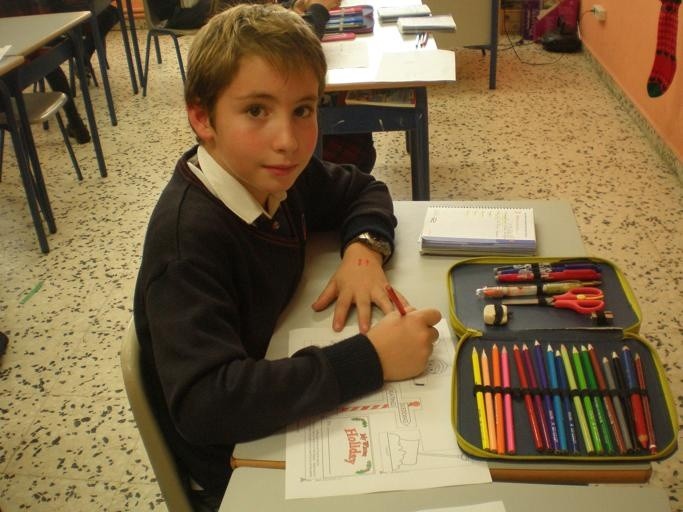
[[0, 1, 138, 252], [112, 311, 191, 506], [140, 6, 212, 91]]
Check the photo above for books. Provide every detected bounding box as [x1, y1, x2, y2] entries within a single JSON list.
[[419, 205, 538, 257], [377, 4, 456, 34]]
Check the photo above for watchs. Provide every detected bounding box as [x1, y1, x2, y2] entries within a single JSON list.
[[340, 232, 392, 264]]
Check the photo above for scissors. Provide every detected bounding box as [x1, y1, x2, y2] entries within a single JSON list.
[[501, 287, 606, 314]]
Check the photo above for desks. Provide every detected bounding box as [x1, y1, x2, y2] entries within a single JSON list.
[[220, 194, 668, 507], [282, 1, 461, 199]]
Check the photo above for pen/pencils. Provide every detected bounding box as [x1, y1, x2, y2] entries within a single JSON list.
[[493, 258, 603, 282], [481, 279, 584, 298], [384, 285, 406, 316], [472, 339, 659, 455]]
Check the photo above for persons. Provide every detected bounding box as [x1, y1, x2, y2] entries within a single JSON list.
[[0, 0, 90, 143], [134, 3, 441, 512], [52, 0, 122, 81], [290, 0, 376, 173]]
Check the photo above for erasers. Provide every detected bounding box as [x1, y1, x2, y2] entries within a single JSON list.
[[483, 304, 508, 325]]
